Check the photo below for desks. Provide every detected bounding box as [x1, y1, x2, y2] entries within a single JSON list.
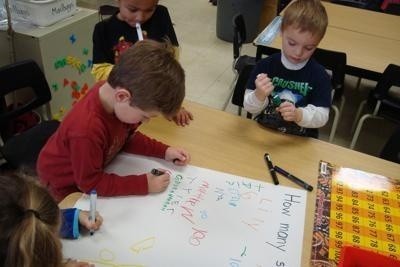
[[253, 16, 400, 89], [278, 0, 400, 40], [48, 97, 400, 267]]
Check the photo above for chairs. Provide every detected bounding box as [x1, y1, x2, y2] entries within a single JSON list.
[[221, 11, 399, 164], [0, 56, 62, 177]]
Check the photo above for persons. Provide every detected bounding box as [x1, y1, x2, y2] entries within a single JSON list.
[[91, 0, 181, 82], [243, 0, 332, 139], [35, 39, 190, 200], [0, 170, 104, 267]]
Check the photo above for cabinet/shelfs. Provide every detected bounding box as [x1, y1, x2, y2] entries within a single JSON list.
[[0, 5, 100, 124]]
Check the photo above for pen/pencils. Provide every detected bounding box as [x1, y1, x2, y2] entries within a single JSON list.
[[136, 22, 143, 41], [90, 190, 97, 235], [274, 165, 313, 191], [256, 73, 281, 106], [264, 153, 279, 185], [150, 168, 164, 176]]
